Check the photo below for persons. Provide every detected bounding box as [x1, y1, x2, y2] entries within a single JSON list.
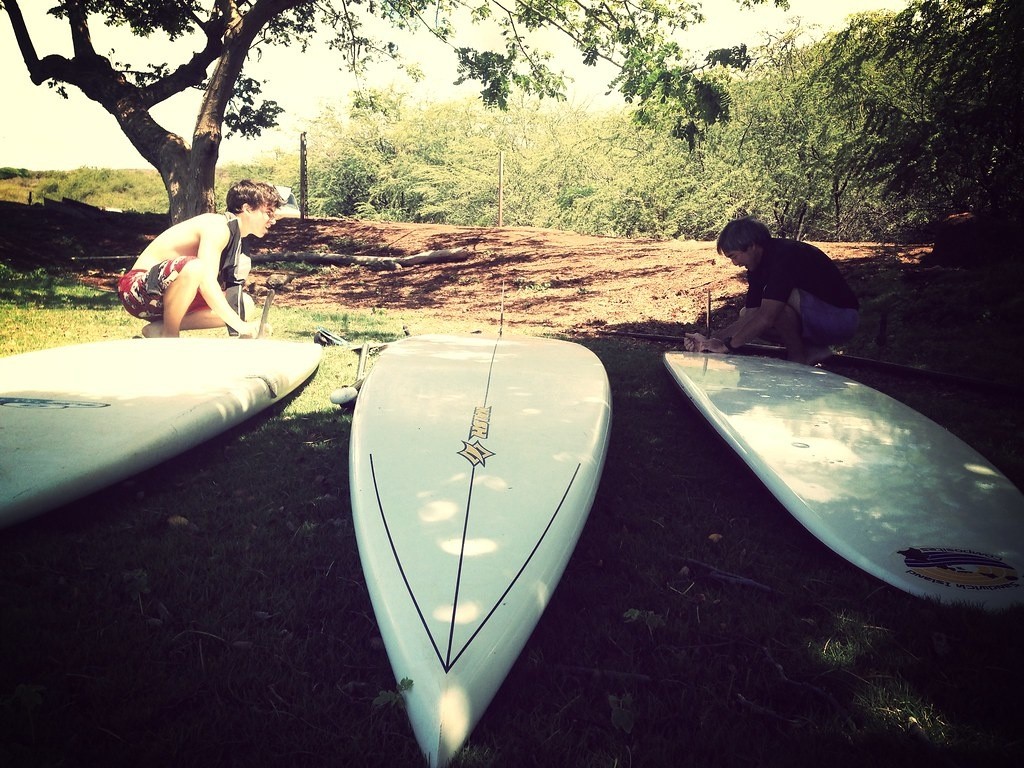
[[683, 219, 862, 367], [117, 180, 283, 339]]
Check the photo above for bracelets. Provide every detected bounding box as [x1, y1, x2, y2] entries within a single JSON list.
[[722, 336, 740, 354]]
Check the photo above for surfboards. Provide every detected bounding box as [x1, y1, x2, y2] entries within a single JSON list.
[[1, 337, 323, 519], [348, 332, 614, 768], [661, 347, 1024, 615]]
